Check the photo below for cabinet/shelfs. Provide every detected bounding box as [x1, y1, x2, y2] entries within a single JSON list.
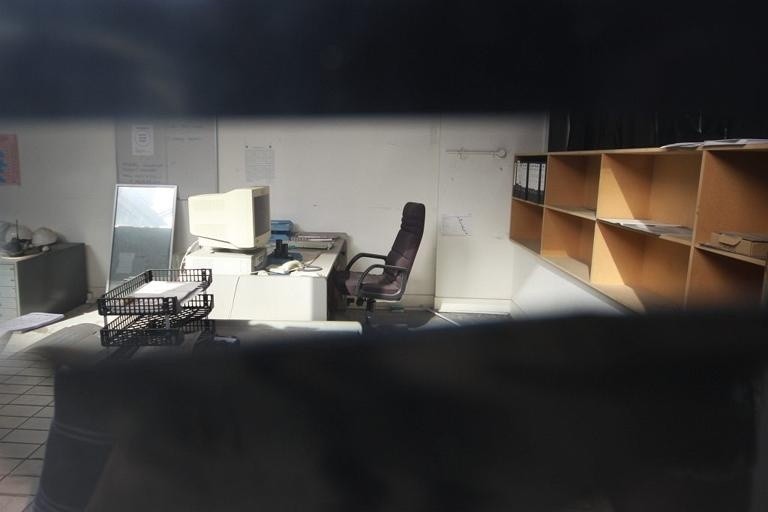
[[97, 269, 216, 366], [0, 243, 87, 334]]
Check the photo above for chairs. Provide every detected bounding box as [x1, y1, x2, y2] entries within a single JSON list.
[[329, 202, 425, 325]]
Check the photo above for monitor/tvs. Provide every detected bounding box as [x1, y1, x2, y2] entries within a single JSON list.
[[188, 186, 272, 254]]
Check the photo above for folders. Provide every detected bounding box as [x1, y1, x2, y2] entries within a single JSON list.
[[512, 157, 549, 205]]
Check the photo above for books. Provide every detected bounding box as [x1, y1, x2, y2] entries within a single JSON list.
[[597, 217, 692, 237], [289, 232, 340, 251]]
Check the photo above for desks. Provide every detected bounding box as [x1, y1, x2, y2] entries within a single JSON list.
[[179, 232, 347, 320]]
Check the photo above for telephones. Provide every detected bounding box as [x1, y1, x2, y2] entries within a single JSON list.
[[265, 259, 303, 275]]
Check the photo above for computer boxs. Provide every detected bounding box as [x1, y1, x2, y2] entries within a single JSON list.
[[185, 247, 268, 276]]
